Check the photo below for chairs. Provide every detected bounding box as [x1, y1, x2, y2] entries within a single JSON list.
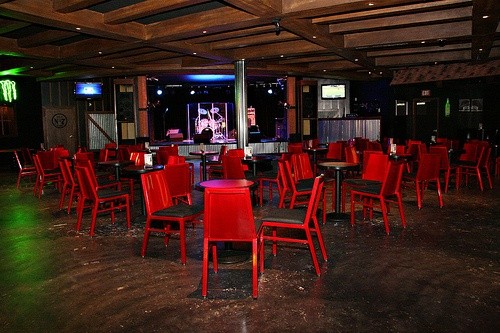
[[203, 185, 262, 302], [139, 174, 203, 262], [261, 172, 328, 275], [10, 145, 136, 233], [262, 137, 500, 235], [149, 143, 249, 179]]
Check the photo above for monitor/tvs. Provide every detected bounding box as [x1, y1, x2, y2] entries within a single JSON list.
[[321, 83, 346, 100], [74, 82, 103, 101]]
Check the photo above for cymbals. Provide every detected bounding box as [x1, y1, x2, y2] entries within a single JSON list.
[[210, 107, 219, 114], [200, 108, 207, 115]]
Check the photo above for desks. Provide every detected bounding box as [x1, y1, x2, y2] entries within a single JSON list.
[[439, 146, 460, 188], [58, 156, 73, 195], [99, 161, 134, 211], [315, 160, 358, 223], [191, 149, 215, 189], [201, 178, 254, 264], [240, 158, 267, 192], [386, 151, 411, 199]]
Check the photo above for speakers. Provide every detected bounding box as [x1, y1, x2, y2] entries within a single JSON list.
[[302, 92, 317, 119], [117, 92, 134, 123]]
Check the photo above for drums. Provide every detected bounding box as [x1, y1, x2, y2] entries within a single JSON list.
[[197, 118, 219, 130]]
